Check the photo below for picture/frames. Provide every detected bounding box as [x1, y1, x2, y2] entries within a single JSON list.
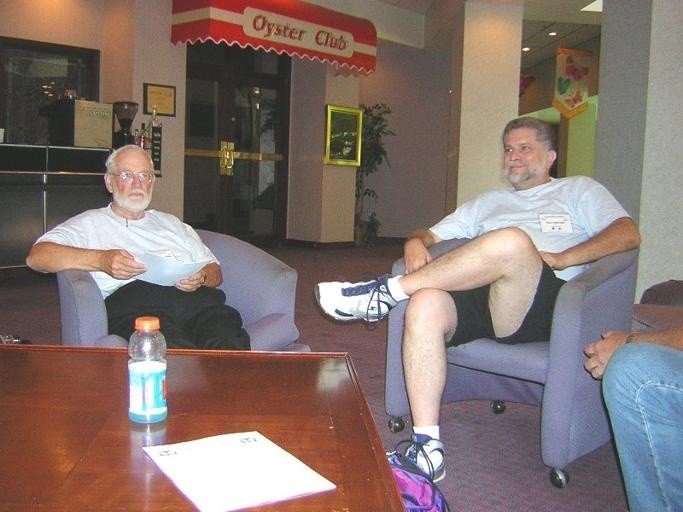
[[143, 83, 176, 116], [325, 104, 362, 166]]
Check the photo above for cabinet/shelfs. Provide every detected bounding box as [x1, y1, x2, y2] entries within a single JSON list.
[[0, 144, 112, 268]]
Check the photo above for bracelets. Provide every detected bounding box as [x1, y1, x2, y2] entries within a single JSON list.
[[199, 268, 209, 288], [624, 326, 640, 345]]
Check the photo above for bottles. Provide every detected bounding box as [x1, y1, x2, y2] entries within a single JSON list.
[[147, 105, 163, 176], [139, 122, 149, 150], [124, 314, 169, 425], [127, 424, 169, 510], [133, 128, 140, 147]]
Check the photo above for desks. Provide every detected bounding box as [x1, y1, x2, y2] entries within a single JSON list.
[[0, 345, 407, 511]]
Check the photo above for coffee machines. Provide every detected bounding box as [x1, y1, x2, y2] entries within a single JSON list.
[[113, 101, 139, 149]]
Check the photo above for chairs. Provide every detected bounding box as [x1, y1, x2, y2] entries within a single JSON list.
[[58, 229, 298, 351], [384, 236, 640, 487]]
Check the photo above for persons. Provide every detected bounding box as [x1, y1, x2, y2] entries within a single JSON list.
[[21, 143, 254, 353], [306, 114, 638, 481], [582, 314, 683, 511]]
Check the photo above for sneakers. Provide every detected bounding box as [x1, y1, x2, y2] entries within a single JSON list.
[[312, 273, 398, 331], [382, 432, 447, 483]]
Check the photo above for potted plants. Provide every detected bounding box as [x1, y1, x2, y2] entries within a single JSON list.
[[354, 102, 397, 245]]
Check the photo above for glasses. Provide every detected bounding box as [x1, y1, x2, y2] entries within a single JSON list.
[[105, 169, 154, 185]]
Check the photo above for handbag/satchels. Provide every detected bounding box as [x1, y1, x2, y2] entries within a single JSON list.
[[383, 448, 451, 512]]
